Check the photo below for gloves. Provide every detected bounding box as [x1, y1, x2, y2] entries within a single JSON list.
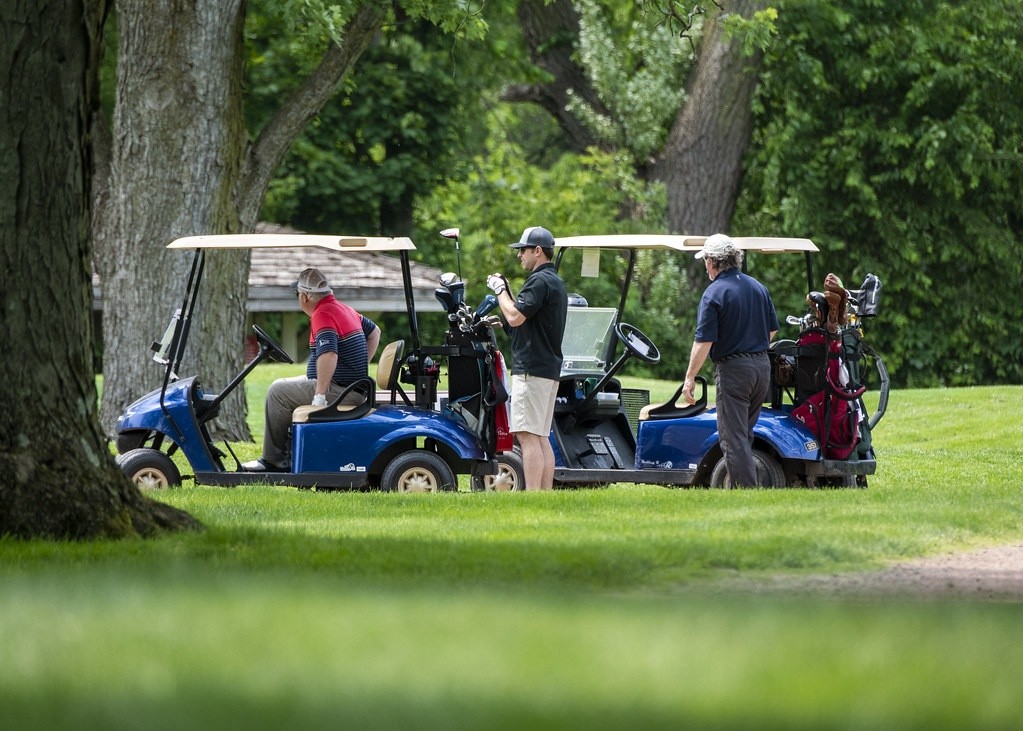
[[486, 273, 510, 295], [311, 394, 328, 406]]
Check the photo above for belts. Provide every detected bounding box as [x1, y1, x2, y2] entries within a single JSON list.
[[715, 349, 768, 366]]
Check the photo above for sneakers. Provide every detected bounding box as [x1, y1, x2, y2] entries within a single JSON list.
[[241, 457, 291, 472]]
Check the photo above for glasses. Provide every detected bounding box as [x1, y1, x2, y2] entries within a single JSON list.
[[519, 247, 537, 254], [294, 288, 307, 297]]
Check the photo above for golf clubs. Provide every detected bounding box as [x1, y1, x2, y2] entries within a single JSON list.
[[433, 227, 500, 335], [785, 272, 861, 336]]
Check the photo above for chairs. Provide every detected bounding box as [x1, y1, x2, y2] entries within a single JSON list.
[[293, 340, 404, 423], [639, 327, 778, 422]]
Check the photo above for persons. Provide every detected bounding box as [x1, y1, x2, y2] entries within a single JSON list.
[[681, 233, 780, 490], [242, 269, 381, 471], [486, 226, 568, 491]]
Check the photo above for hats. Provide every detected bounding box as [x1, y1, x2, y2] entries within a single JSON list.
[[290, 268, 329, 293], [694, 232, 736, 260], [509, 225, 555, 249]]
